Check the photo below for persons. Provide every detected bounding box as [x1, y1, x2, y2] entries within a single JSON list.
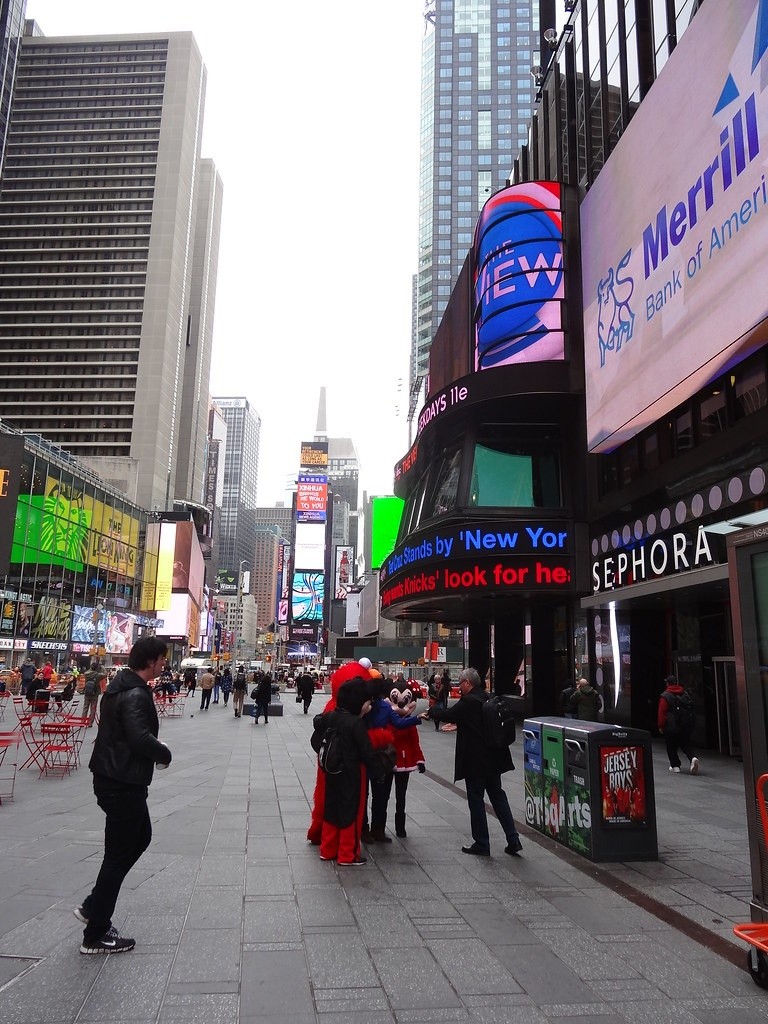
[[56, 675, 77, 712], [254, 670, 271, 723], [200, 666, 258, 718], [82, 663, 106, 727], [26, 672, 50, 700], [395, 672, 406, 682], [156, 665, 196, 703], [17, 657, 37, 695], [570, 679, 600, 722], [70, 666, 79, 690], [427, 671, 452, 732], [73, 638, 172, 954], [361, 696, 428, 844], [40, 660, 54, 687], [658, 675, 699, 774], [432, 667, 524, 855], [283, 670, 325, 714]]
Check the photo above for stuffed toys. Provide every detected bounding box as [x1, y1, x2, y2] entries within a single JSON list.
[[309, 657, 426, 866]]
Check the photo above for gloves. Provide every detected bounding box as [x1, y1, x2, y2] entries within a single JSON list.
[[417, 762, 426, 773]]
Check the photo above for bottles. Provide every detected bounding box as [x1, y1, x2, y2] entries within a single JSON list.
[[156, 742, 169, 770]]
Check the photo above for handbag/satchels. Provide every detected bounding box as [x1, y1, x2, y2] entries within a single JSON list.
[[250, 687, 261, 699]]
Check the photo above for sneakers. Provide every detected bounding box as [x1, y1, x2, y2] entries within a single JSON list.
[[74, 905, 119, 937], [339, 856, 367, 865], [320, 856, 326, 859], [668, 766, 681, 773], [690, 757, 699, 774], [79, 930, 136, 954]]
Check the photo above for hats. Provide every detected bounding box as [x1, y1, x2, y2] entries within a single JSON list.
[[663, 676, 677, 684]]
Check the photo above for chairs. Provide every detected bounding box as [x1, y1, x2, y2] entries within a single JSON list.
[[153, 690, 188, 725], [0, 691, 101, 807]]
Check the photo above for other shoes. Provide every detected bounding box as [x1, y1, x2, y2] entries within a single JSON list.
[[265, 721, 268, 723], [362, 824, 373, 844], [370, 831, 392, 844], [505, 838, 522, 854], [238, 716, 241, 717], [462, 842, 490, 856], [235, 709, 238, 717], [255, 717, 258, 724]]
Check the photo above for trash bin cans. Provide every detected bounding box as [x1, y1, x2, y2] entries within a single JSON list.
[[523, 715, 658, 864]]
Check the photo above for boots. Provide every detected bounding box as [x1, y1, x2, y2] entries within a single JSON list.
[[395, 812, 406, 838]]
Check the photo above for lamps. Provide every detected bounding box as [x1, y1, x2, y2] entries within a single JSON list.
[[529, 64, 543, 87], [564, 0, 579, 12], [543, 27, 558, 51]]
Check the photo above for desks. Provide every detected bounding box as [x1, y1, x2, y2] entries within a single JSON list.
[[27, 701, 62, 731], [47, 722, 86, 769]]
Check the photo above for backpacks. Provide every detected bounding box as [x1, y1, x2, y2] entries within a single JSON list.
[[233, 676, 246, 691], [463, 694, 516, 753], [660, 691, 696, 731], [84, 673, 98, 700], [317, 714, 352, 775]]
